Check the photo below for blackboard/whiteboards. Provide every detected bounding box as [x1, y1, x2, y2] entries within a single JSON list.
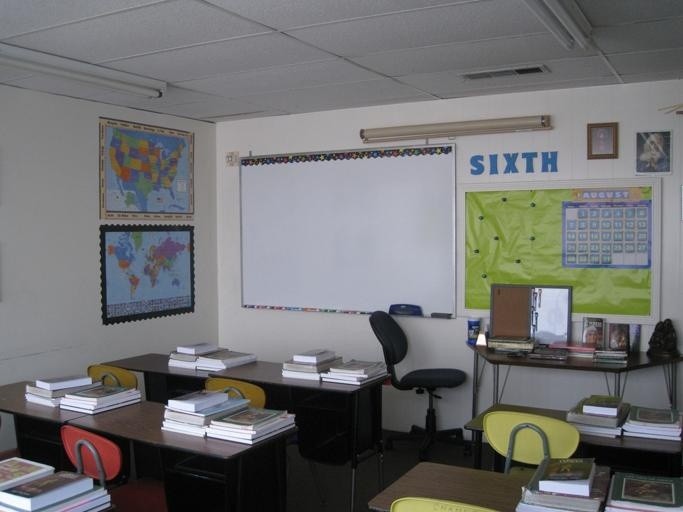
[[239, 143, 457, 320]]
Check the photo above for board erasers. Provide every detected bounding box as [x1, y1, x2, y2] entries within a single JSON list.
[[432, 312, 452, 319]]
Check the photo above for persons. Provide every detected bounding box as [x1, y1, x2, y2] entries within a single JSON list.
[[609, 330, 628, 352], [583, 326, 599, 347], [638, 134, 669, 173]]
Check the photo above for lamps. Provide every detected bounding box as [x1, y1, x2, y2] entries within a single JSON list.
[[0, 41, 167, 100], [360, 115, 553, 143], [526, 0, 593, 51]]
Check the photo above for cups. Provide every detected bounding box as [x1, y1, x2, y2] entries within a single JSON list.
[[468, 320, 481, 345]]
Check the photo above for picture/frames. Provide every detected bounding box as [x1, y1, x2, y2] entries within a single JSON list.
[[587, 122, 618, 159]]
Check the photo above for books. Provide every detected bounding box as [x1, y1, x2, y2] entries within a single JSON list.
[[1, 455, 112, 512], [281, 349, 389, 385], [167, 342, 258, 372], [514, 457, 683, 512], [486, 341, 629, 365], [161, 390, 296, 444], [26, 374, 143, 415], [564, 393, 682, 443]]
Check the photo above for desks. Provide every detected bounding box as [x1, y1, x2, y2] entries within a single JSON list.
[[368, 462, 683, 511], [100, 353, 394, 511], [463, 404, 683, 471], [466, 336, 682, 469], [0, 380, 298, 512]]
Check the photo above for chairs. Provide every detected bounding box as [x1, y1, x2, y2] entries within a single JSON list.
[[370, 310, 467, 462], [206, 378, 266, 410], [87, 365, 137, 391], [59, 425, 165, 511], [483, 410, 580, 473], [389, 497, 494, 512]]
